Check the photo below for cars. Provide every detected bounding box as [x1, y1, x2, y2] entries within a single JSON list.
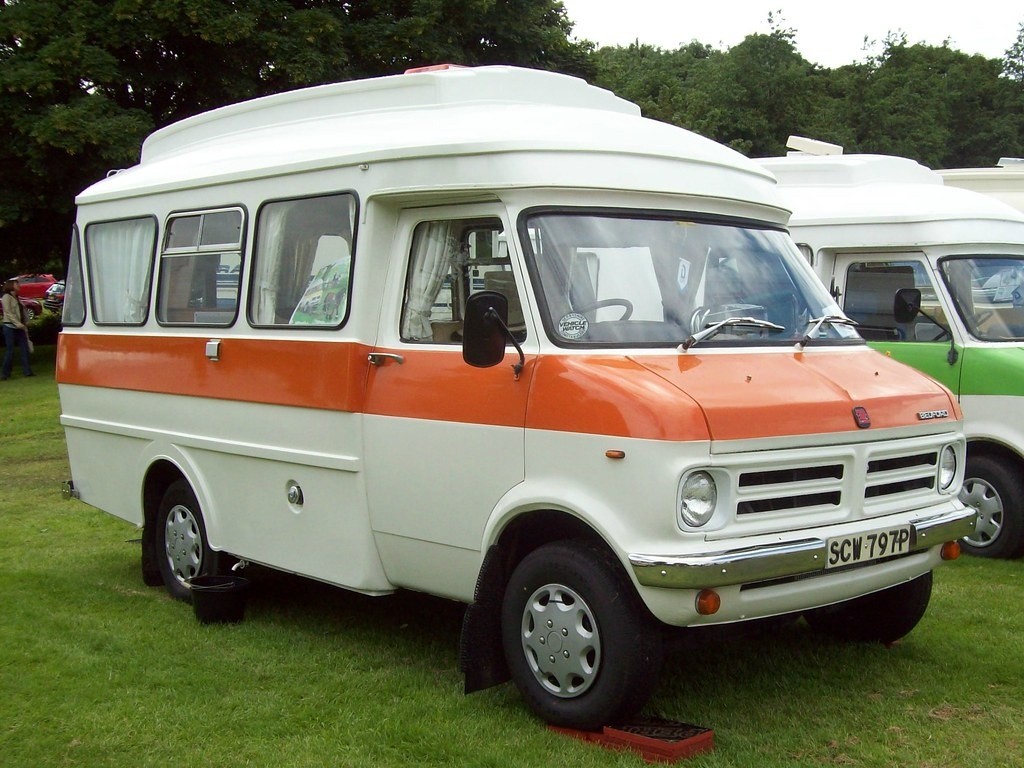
[[0, 291, 43, 322]]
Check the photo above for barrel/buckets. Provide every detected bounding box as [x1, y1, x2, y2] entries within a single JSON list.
[[184, 574, 248, 625]]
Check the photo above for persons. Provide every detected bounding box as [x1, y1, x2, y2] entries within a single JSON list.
[[0, 277, 36, 381]]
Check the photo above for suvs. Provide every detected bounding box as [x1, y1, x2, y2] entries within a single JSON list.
[[43, 280, 65, 314]]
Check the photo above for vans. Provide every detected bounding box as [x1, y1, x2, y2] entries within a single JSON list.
[[0, 274, 57, 299], [743, 137, 1023, 559], [924, 157, 1024, 219], [53, 64, 979, 734]]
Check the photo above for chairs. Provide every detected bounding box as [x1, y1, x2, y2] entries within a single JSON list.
[[484, 271, 526, 331]]
